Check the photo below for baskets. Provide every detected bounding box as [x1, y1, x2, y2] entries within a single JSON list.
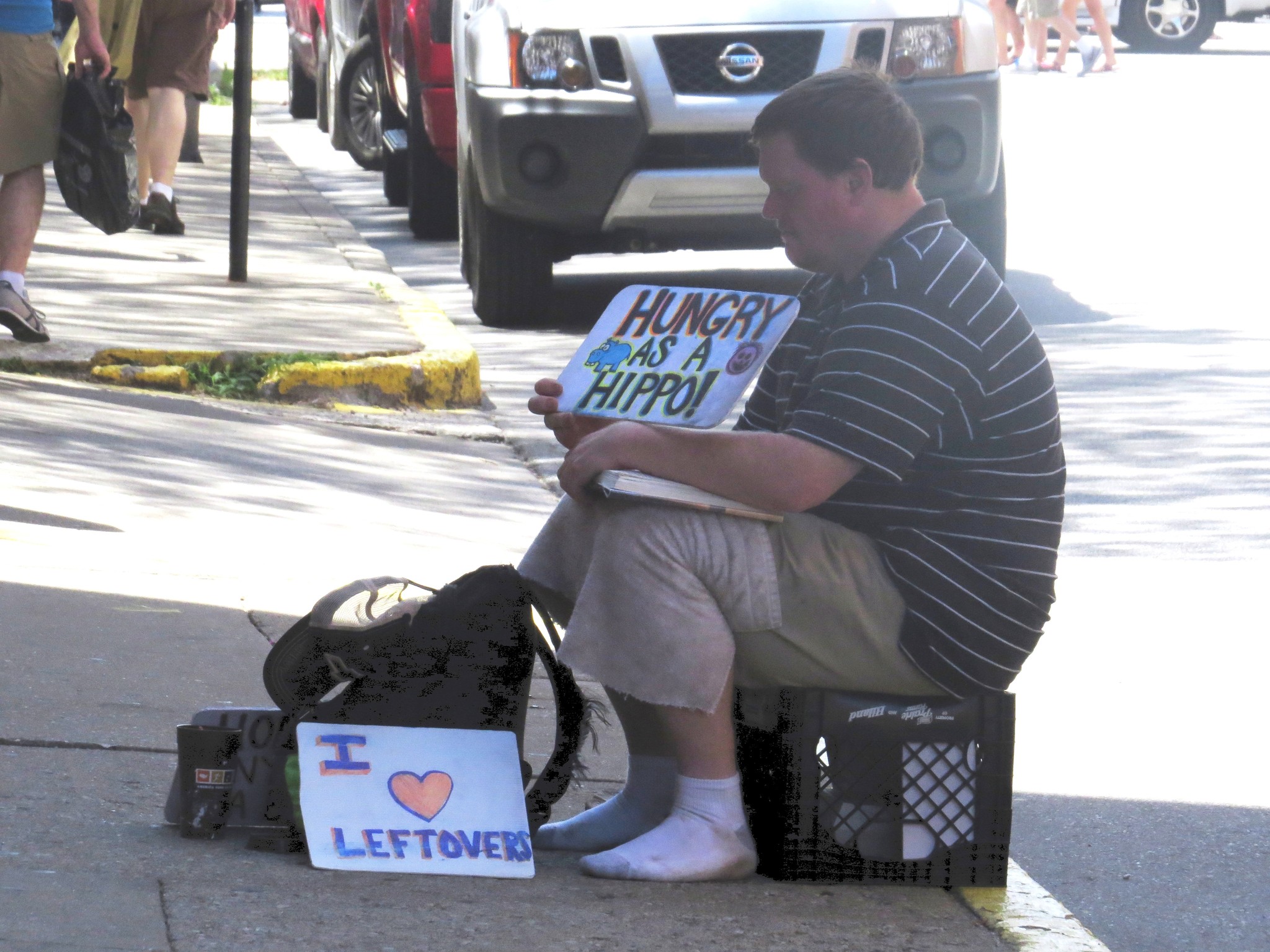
[[728, 679, 1017, 891]]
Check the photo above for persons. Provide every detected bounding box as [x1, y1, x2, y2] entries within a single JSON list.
[[530, 69, 1068, 878], [0, 0, 237, 343], [987, 0, 1119, 75]]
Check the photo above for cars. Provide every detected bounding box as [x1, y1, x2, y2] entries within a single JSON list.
[[1018, 0, 1270, 51]]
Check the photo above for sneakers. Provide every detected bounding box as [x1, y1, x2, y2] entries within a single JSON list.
[[0, 281, 51, 343]]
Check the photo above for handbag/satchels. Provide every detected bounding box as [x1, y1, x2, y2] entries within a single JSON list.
[[51, 62, 143, 236]]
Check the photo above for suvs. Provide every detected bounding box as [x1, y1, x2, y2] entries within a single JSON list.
[[284, 0, 1006, 326]]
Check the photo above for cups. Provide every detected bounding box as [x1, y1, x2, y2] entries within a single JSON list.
[[176, 724, 243, 840]]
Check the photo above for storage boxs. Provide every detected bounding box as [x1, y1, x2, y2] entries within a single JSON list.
[[735, 688, 1016, 887]]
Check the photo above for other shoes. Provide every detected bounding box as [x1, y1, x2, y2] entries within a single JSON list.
[[144, 191, 186, 234], [1078, 46, 1103, 77], [127, 201, 154, 232]]
